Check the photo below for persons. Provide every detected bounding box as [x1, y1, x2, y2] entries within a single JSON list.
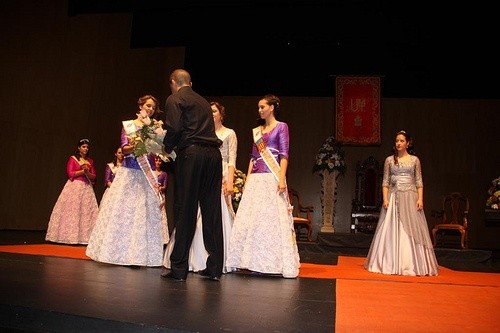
[[161, 69, 224, 282], [85, 95, 169, 267], [45, 139, 99, 245], [364, 131, 440, 276], [224, 96, 301, 278], [163, 102, 240, 273]]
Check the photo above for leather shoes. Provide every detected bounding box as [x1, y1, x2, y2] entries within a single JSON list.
[[160, 270, 187, 281], [198, 269, 221, 280]]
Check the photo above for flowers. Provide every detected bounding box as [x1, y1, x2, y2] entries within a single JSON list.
[[233, 169, 246, 208], [130, 110, 177, 163], [481, 175, 500, 210], [312, 137, 346, 178]]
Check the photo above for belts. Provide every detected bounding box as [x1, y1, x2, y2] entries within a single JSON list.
[[190, 141, 218, 148]]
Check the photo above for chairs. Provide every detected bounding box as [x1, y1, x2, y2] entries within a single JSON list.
[[432, 193, 471, 248], [350, 156, 383, 234], [285, 187, 315, 241]]
